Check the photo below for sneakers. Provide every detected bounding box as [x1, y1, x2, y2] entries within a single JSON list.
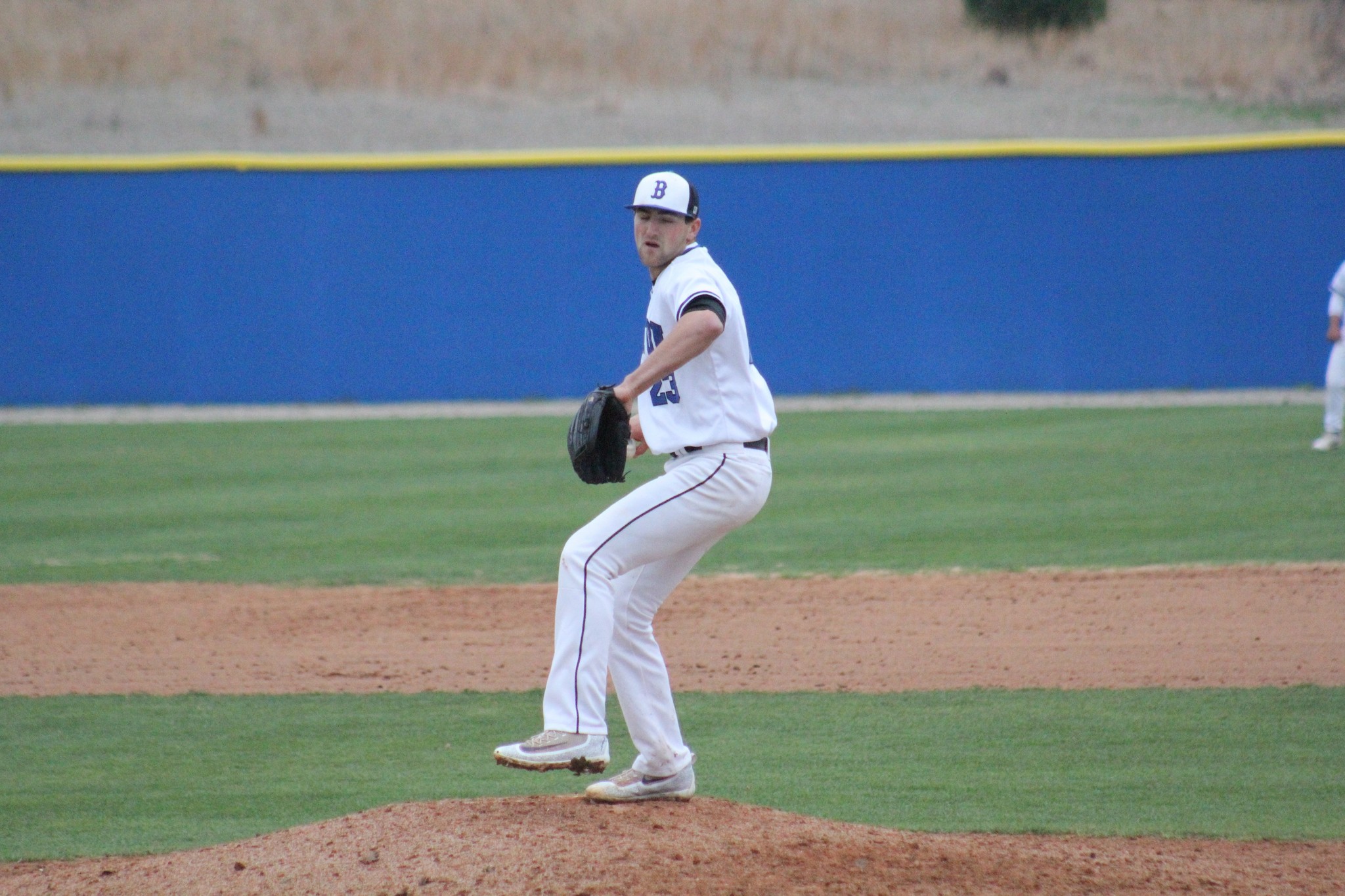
[[586, 759, 695, 803], [493, 725, 613, 773]]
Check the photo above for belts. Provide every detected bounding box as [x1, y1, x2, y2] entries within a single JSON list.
[[670, 437, 769, 458]]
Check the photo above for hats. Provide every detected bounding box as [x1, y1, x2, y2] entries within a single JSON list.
[[624, 170, 698, 221]]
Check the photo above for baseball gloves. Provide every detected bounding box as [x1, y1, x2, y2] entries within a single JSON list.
[[565, 383, 635, 487]]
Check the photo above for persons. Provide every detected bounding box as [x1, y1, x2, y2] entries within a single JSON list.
[[494, 171, 779, 804], [1313, 259, 1345, 451]]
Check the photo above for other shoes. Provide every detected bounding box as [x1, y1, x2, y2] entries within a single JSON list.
[[1313, 431, 1341, 451]]
